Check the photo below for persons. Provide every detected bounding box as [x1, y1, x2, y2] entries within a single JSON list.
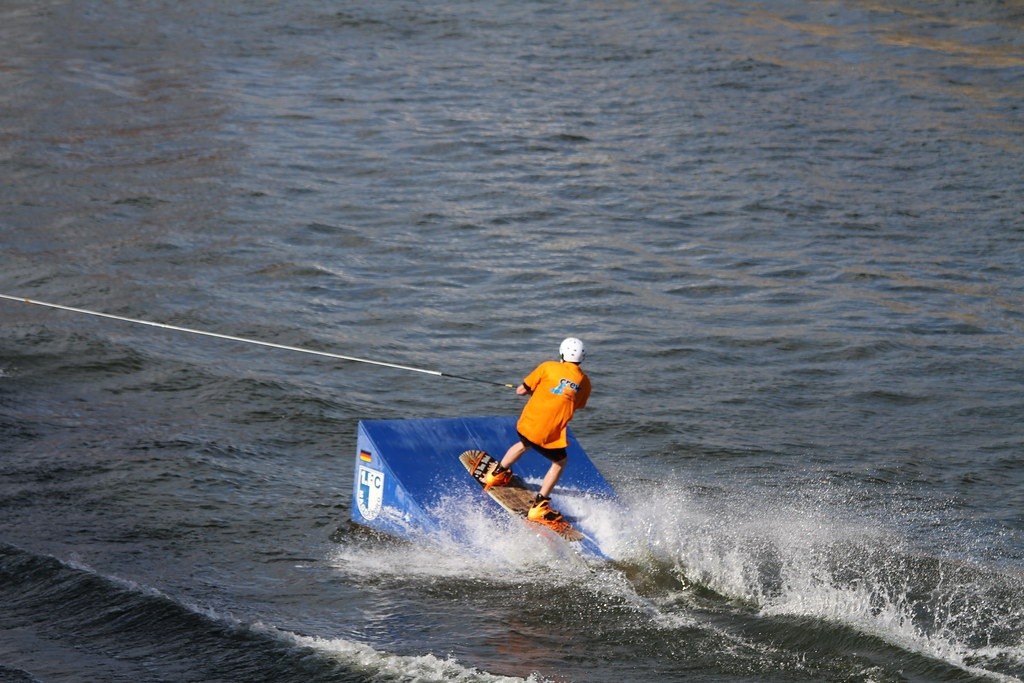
[[488, 338, 592, 523]]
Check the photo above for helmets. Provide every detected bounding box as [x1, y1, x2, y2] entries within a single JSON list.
[[559, 337, 585, 362]]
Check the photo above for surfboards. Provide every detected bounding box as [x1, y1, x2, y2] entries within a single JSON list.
[[459, 447, 584, 545]]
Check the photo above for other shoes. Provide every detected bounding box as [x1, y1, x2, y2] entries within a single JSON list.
[[484, 460, 513, 485], [527, 494, 562, 522]]
